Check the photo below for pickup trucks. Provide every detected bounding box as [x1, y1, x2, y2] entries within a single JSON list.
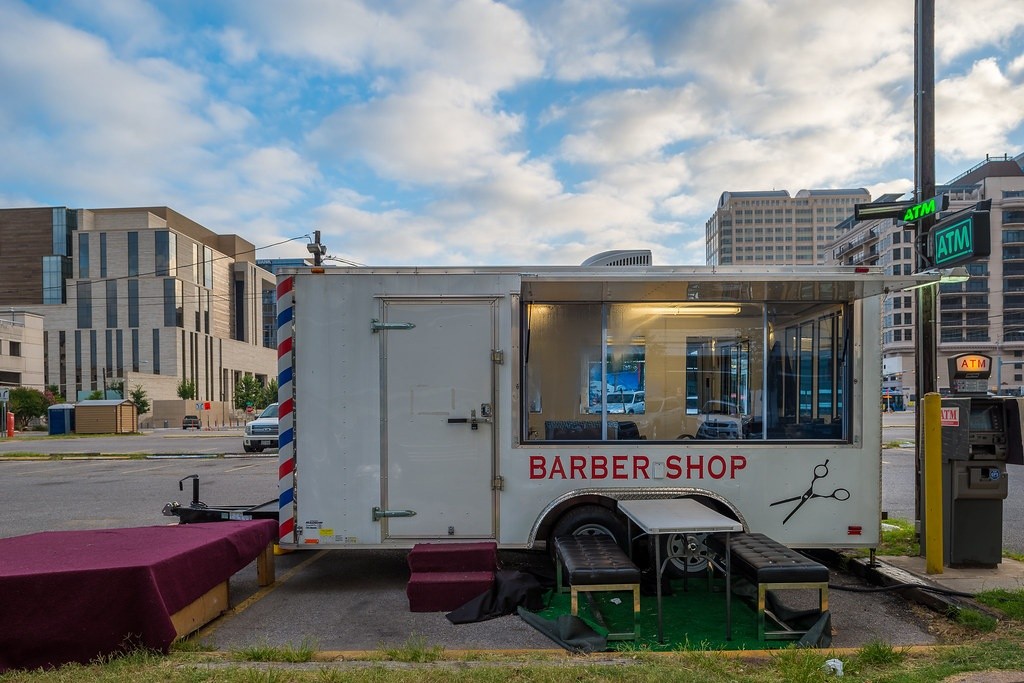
[[183, 415, 201, 429]]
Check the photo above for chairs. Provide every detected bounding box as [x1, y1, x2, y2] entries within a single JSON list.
[[618, 421, 646, 440], [677, 420, 746, 440]]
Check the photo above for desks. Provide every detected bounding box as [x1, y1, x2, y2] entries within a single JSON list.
[[617, 499, 743, 645]]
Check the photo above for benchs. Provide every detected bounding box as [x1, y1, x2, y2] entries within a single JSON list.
[[553, 533, 641, 643], [702, 532, 830, 641]]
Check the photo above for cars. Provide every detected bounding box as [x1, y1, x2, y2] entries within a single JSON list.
[[685, 396, 698, 415], [696, 400, 753, 440], [590, 390, 645, 414], [242, 402, 278, 453]]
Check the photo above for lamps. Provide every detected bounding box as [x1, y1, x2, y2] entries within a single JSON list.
[[627, 306, 743, 315]]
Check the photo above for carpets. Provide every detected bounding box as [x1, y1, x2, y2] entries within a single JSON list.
[[516, 578, 832, 655]]
